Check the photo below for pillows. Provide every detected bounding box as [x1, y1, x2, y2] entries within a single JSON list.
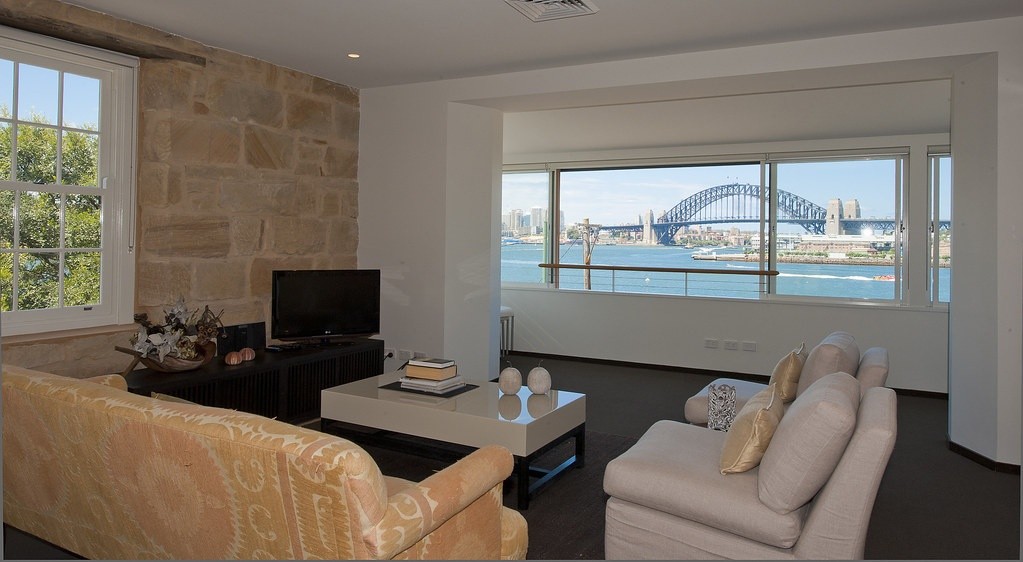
[[719, 381, 784, 475], [768, 341, 808, 403]]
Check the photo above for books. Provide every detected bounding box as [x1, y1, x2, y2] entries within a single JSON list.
[[398, 354, 465, 395]]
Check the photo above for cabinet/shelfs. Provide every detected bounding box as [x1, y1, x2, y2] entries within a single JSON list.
[[111, 337, 386, 428]]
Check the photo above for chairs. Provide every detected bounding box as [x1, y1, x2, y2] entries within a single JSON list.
[[500, 307, 515, 357]]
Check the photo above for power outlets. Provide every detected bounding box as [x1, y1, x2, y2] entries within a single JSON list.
[[742, 342, 757, 352], [398, 350, 411, 361], [413, 352, 427, 358], [723, 340, 737, 350], [704, 339, 718, 348], [384, 348, 397, 359]]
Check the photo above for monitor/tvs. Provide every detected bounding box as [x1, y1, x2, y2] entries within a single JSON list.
[[271, 269, 380, 341]]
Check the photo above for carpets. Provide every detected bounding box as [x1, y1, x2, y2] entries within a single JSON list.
[[296, 418, 638, 560]]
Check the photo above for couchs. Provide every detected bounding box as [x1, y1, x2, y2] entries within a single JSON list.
[[684, 330, 889, 430], [602, 387, 899, 560], [0, 363, 530, 561]]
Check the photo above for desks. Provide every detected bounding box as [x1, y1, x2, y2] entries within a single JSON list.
[[320, 370, 587, 509]]
